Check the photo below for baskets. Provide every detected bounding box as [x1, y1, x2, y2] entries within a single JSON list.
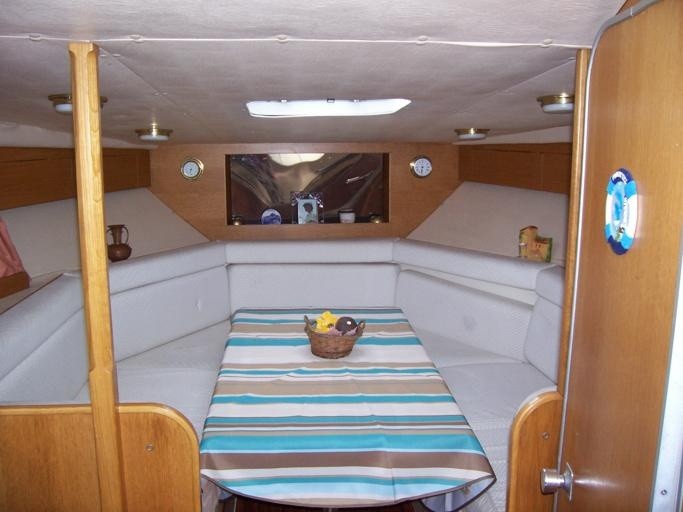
[[303, 314, 366, 360]]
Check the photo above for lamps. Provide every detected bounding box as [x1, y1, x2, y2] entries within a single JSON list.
[[48, 93, 107, 114], [535, 92, 573, 113], [134, 128, 173, 141], [454, 128, 489, 139]]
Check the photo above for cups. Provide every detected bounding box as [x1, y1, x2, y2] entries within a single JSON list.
[[338, 209, 355, 223]]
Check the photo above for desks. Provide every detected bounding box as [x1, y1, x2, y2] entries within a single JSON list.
[[197, 306, 497, 510]]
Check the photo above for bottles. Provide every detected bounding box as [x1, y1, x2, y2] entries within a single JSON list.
[[106, 224, 131, 261], [517, 227, 528, 260]]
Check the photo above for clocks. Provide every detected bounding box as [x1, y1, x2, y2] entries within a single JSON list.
[[178, 155, 204, 184], [409, 155, 433, 179]]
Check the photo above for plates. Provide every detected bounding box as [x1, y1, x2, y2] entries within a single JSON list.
[[259, 208, 281, 224]]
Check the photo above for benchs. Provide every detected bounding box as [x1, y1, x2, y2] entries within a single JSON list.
[[1, 237, 569, 512]]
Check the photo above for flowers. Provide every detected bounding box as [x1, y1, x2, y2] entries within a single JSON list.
[[312, 310, 338, 336]]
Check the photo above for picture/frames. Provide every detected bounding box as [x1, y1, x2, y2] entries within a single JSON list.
[[289, 190, 325, 225]]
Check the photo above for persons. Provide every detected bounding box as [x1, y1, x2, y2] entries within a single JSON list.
[[302, 203, 317, 224]]
